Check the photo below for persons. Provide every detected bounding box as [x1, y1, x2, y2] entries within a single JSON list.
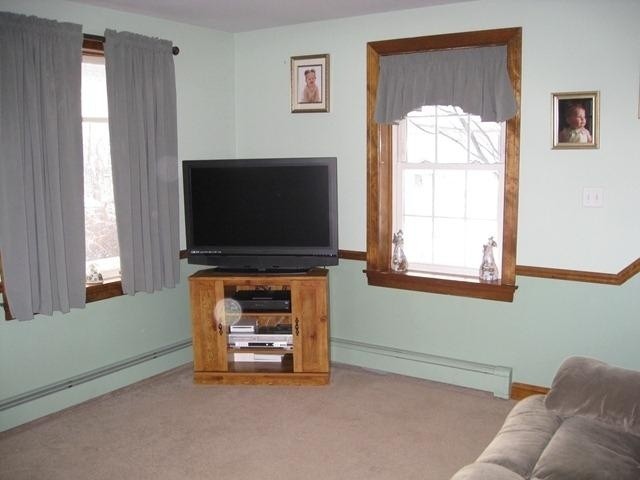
[[301, 68, 320, 102], [559, 102, 592, 142]]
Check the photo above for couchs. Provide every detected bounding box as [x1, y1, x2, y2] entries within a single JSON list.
[[453, 359, 640, 479]]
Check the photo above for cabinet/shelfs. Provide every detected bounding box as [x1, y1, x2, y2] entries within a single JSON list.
[[188, 269, 331, 386]]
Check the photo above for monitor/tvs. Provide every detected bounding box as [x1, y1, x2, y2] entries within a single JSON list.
[[182, 157, 339, 273]]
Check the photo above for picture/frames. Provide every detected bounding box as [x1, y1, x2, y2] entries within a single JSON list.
[[290, 54, 330, 114], [550, 91, 600, 149]]
